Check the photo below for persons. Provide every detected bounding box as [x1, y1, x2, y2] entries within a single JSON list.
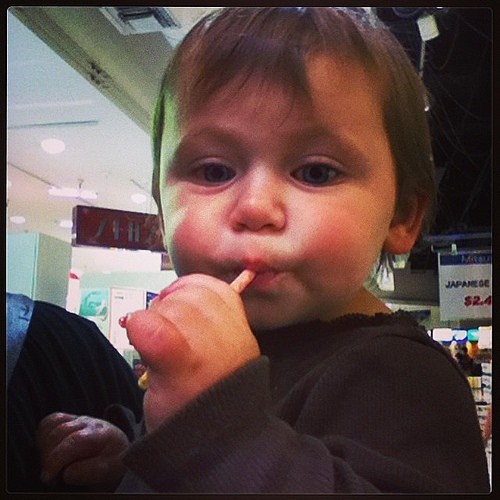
[[36, 7, 490, 493], [449, 341, 482, 376]]
